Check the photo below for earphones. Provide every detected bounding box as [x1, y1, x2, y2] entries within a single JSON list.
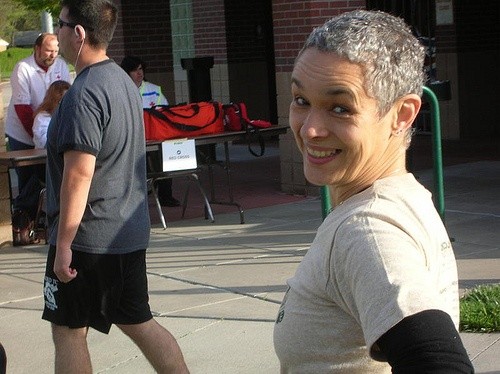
[[75, 28, 80, 36]]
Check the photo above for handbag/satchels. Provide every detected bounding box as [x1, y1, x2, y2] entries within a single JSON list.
[[224, 101, 249, 132], [140, 99, 225, 142]]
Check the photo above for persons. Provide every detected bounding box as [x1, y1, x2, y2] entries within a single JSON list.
[[13, 80, 72, 244], [5, 34, 69, 244], [121, 57, 181, 208], [42, 0, 190, 374], [274, 11, 475, 374]]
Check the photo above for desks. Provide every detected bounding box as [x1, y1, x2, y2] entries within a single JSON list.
[[0, 125, 288, 248]]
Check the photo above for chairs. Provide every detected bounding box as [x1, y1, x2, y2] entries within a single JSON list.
[[145, 155, 216, 229]]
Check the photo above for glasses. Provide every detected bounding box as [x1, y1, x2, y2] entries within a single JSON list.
[[57, 18, 76, 29]]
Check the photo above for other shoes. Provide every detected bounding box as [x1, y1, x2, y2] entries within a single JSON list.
[[157, 197, 181, 207]]
